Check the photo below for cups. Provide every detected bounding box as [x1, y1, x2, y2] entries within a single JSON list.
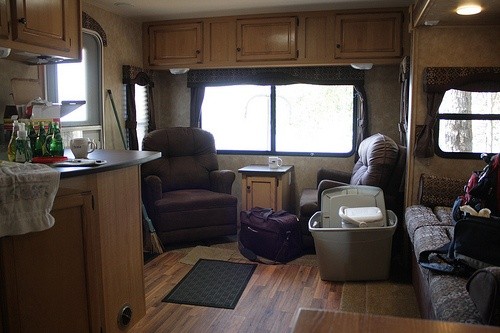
[[268, 157, 283, 169], [70, 138, 96, 158]]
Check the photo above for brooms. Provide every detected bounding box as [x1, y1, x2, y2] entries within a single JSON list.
[[107, 89, 164, 254]]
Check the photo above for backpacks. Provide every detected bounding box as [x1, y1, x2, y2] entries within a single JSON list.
[[452, 153, 500, 225]]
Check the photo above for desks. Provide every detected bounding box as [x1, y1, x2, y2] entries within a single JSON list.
[[291, 308, 500, 333]]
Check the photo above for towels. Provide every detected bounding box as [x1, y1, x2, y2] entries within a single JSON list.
[[0, 159, 61, 237]]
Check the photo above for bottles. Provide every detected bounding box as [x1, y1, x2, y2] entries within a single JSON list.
[[7, 120, 64, 162]]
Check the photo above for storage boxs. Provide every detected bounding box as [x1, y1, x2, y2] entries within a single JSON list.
[[307, 184, 398, 282]]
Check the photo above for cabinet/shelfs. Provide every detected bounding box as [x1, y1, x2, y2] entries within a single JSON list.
[[0, 0, 83, 65], [142, 6, 412, 72], [238, 164, 293, 214]]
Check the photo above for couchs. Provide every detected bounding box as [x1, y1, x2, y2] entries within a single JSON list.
[[404, 173, 500, 326], [298, 132, 402, 254], [141, 127, 238, 257]]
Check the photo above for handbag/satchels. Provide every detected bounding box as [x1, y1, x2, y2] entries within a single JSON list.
[[420, 197, 500, 275], [236, 206, 302, 264]]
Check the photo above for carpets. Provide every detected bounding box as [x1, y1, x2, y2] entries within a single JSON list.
[[338, 280, 422, 319], [161, 259, 259, 310]]
